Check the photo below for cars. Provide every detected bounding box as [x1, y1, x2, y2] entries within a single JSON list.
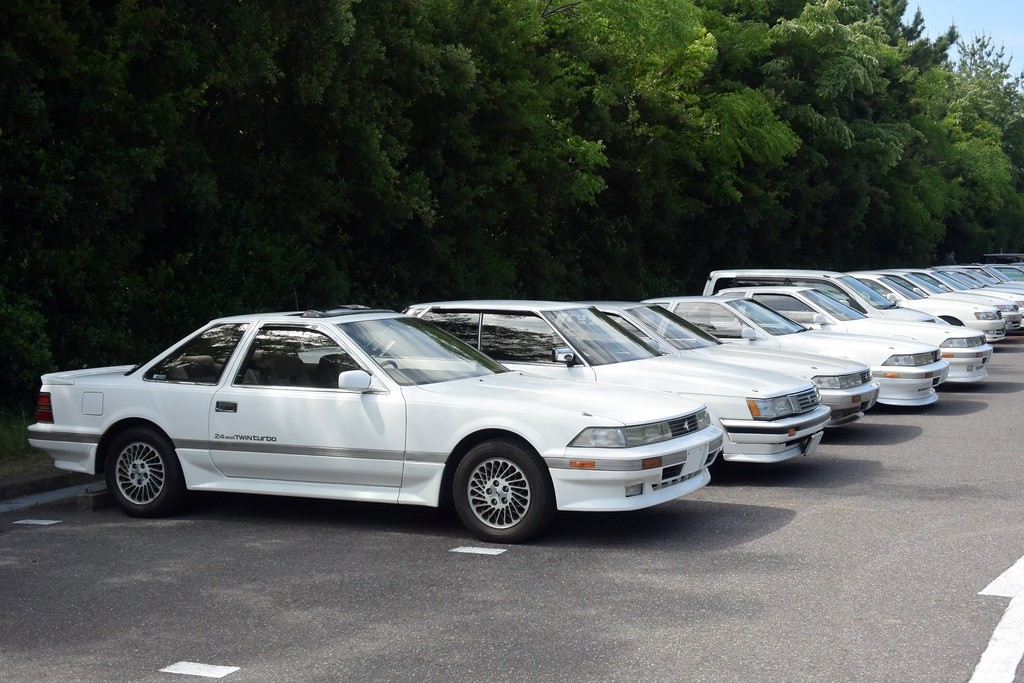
[[254, 300, 834, 465], [556, 251, 1024, 428], [26, 303, 725, 545]]
